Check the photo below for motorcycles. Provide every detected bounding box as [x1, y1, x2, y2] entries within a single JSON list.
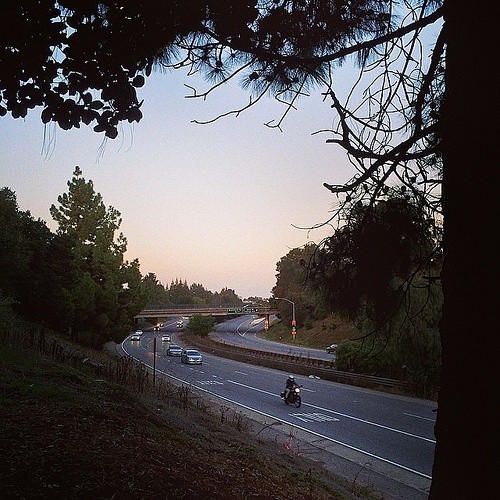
[[280, 384, 303, 408]]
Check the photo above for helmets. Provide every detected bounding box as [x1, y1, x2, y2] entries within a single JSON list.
[[288, 375, 295, 381]]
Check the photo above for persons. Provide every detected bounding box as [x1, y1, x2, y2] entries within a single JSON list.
[[284, 374, 301, 399]]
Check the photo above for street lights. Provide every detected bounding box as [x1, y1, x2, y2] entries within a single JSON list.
[[275, 297, 295, 341]]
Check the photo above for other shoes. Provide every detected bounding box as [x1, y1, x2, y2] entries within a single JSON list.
[[286, 398, 289, 401]]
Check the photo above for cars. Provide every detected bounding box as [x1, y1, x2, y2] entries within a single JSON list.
[[166, 345, 184, 356], [153, 326, 160, 331], [161, 334, 170, 342], [175, 320, 184, 323], [177, 323, 183, 328], [157, 322, 163, 327], [130, 334, 140, 341], [135, 330, 144, 336], [181, 349, 203, 365], [327, 343, 338, 354]]
